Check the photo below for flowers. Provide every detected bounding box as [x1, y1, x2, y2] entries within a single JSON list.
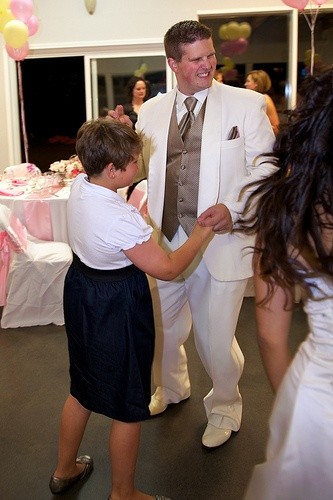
[[50, 156, 86, 187]]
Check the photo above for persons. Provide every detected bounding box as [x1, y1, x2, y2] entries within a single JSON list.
[[241, 67, 333, 500], [121, 77, 151, 129], [109, 21, 277, 447], [50, 117, 214, 500], [243, 70, 279, 134]]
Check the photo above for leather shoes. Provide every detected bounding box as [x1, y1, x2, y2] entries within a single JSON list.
[[49, 455, 93, 495], [202, 424, 232, 447], [148, 395, 190, 415], [108, 493, 170, 500]]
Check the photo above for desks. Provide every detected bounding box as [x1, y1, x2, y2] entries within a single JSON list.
[[0, 172, 127, 242]]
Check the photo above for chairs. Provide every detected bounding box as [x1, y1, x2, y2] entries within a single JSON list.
[[122, 180, 148, 221], [0, 204, 73, 329]]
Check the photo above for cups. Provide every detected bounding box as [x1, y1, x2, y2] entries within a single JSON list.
[[44, 172, 54, 185], [55, 172, 65, 187]]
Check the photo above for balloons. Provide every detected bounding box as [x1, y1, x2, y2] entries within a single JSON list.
[[0, 0, 18, 34], [85, 0, 95, 14], [10, 0, 32, 23], [4, 21, 29, 47], [219, 21, 251, 56], [304, 48, 322, 70], [5, 42, 30, 59], [221, 57, 238, 77], [23, 16, 38, 36], [134, 63, 148, 78], [283, 0, 326, 9]]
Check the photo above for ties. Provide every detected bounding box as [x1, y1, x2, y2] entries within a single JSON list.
[[178, 97, 198, 143]]
